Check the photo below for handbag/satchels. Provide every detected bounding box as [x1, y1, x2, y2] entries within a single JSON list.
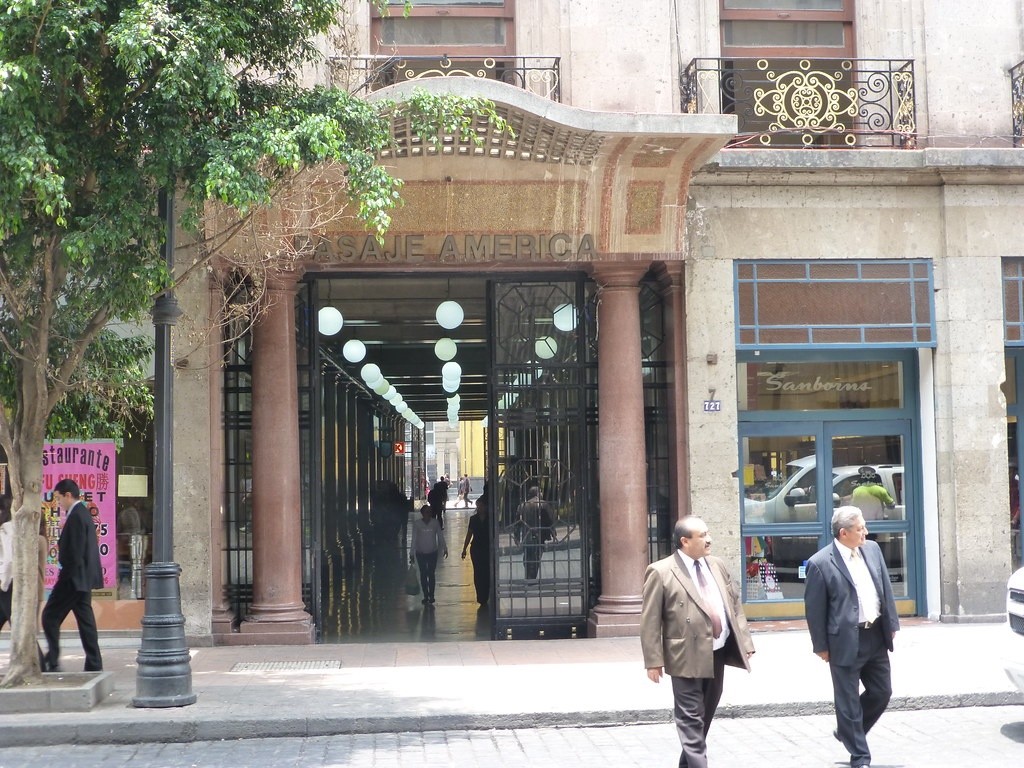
[[404, 560, 420, 595], [459, 494, 464, 500]]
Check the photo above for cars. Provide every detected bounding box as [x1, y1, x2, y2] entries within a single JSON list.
[[1006, 566, 1024, 643], [742, 455, 906, 571]]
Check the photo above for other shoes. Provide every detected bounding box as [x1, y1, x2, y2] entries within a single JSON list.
[[422, 599, 428, 604], [833, 729, 842, 742], [429, 599, 435, 603]]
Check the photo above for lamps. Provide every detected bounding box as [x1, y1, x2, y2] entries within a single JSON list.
[[554, 297, 579, 331], [434, 330, 457, 361], [535, 334, 558, 359], [482, 361, 543, 428], [361, 363, 380, 382], [395, 401, 408, 413], [381, 385, 396, 401], [401, 408, 425, 430], [366, 374, 384, 390], [389, 392, 402, 406], [442, 361, 463, 393], [342, 327, 366, 363], [447, 394, 461, 428], [435, 276, 464, 330], [373, 378, 389, 396], [319, 279, 344, 336]]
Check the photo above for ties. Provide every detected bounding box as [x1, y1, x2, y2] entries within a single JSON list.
[[694, 560, 722, 639], [851, 549, 858, 557]]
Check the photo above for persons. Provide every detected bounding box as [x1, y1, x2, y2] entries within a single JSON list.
[[853, 472, 895, 554], [1010, 467, 1020, 527], [409, 505, 449, 604], [439, 476, 449, 511], [805, 506, 900, 768], [428, 483, 444, 530], [119, 496, 141, 535], [461, 498, 489, 607], [453, 473, 473, 507], [42, 479, 104, 671], [1, 495, 47, 671], [512, 487, 556, 580], [639, 516, 756, 768]]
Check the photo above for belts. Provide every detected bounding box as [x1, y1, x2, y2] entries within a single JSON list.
[[858, 622, 880, 629]]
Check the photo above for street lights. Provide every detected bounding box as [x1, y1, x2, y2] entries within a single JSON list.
[[130, 10, 199, 707]]
